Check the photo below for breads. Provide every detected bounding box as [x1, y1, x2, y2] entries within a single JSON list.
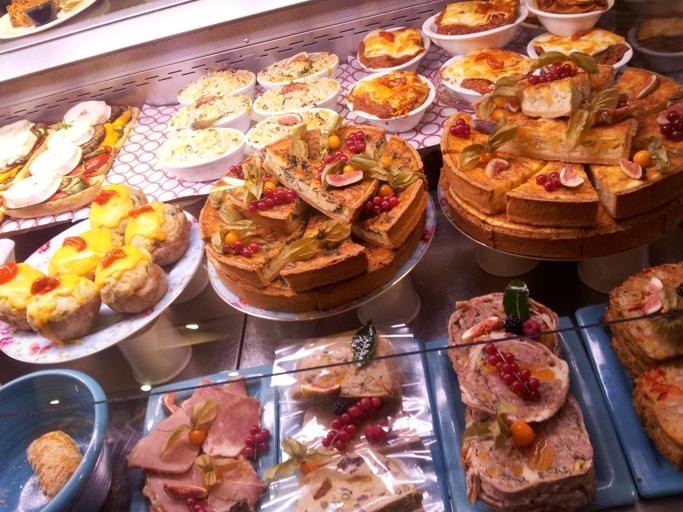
[[448, 281, 600, 511], [1, 98, 139, 221], [606, 262, 683, 466], [0, 186, 190, 344], [8, 1, 64, 30], [199, 122, 431, 312], [286, 328, 423, 511], [27, 426, 84, 499], [439, 54, 682, 257]]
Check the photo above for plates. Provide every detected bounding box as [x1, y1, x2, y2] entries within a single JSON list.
[[421, 318, 638, 510], [574, 300, 682, 499], [206, 190, 440, 321], [275, 336, 450, 512], [0, 208, 204, 365], [435, 183, 654, 262], [0, 1, 98, 40], [130, 362, 278, 512]]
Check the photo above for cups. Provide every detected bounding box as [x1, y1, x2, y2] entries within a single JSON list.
[[114, 312, 192, 385], [474, 240, 540, 278], [576, 244, 652, 295], [356, 275, 422, 332], [169, 262, 210, 307]]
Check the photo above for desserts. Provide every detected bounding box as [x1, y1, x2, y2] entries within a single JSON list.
[[635, 10, 683, 54], [436, 0, 522, 37], [358, 28, 424, 68], [536, 0, 610, 15], [440, 47, 530, 92], [534, 29, 627, 69], [348, 70, 429, 118]]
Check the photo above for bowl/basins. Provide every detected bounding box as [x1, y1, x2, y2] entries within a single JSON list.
[[345, 73, 436, 134], [437, 51, 532, 103], [355, 25, 429, 73], [421, 4, 531, 56], [523, 0, 616, 37], [628, 16, 683, 73], [525, 32, 633, 78], [0, 368, 113, 510], [159, 2, 341, 182]]
